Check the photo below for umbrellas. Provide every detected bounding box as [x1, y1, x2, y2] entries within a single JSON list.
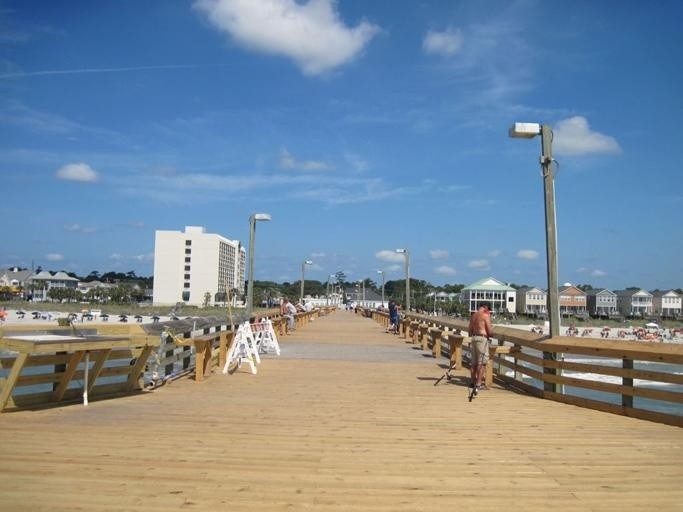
[[15, 311, 194, 323]]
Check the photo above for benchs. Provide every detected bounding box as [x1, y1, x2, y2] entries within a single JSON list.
[[0, 326, 161, 413], [164, 308, 319, 384]]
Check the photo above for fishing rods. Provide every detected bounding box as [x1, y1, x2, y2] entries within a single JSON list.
[[434, 351, 471, 386], [468, 336, 493, 402]]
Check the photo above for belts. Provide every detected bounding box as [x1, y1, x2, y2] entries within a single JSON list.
[[473, 334, 487, 337]]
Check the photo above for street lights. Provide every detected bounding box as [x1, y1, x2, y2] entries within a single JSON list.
[[326, 275, 334, 305], [377, 271, 384, 306], [509, 123, 563, 393], [247, 213, 272, 314], [300, 260, 312, 304], [397, 248, 410, 313], [359, 280, 365, 306]]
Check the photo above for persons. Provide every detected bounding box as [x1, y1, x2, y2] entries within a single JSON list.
[[0, 306, 8, 325], [386, 299, 402, 335], [302, 301, 314, 322], [280, 296, 296, 335], [350, 303, 354, 312], [295, 300, 306, 312], [286, 301, 297, 314], [533, 326, 676, 343], [467, 301, 495, 390]]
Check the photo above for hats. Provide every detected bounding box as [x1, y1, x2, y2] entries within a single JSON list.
[[480, 302, 491, 310]]
